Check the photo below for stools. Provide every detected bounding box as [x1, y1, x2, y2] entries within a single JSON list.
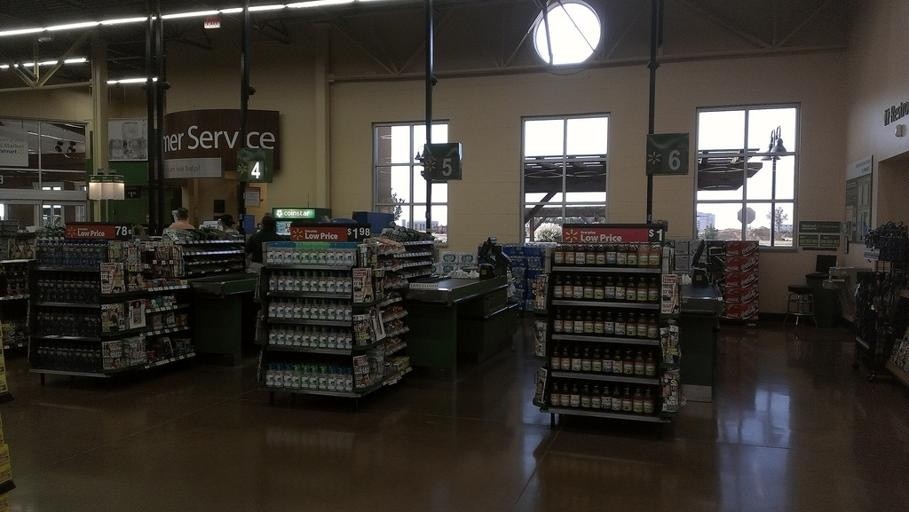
[[782, 284, 819, 330]]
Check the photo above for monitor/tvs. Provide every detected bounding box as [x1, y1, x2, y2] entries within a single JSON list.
[[240, 214, 255, 232], [816, 255, 839, 273], [478, 237, 497, 256]]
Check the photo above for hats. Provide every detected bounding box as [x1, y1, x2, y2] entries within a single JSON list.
[[175, 208, 189, 217], [220, 214, 236, 224]]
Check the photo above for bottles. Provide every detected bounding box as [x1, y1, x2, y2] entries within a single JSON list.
[[264, 249, 357, 392], [1, 267, 28, 296], [32, 236, 106, 373], [548, 240, 662, 413]]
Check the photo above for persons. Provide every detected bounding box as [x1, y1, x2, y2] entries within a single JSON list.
[[169, 208, 195, 229], [244, 216, 284, 264], [221, 215, 239, 232]]
